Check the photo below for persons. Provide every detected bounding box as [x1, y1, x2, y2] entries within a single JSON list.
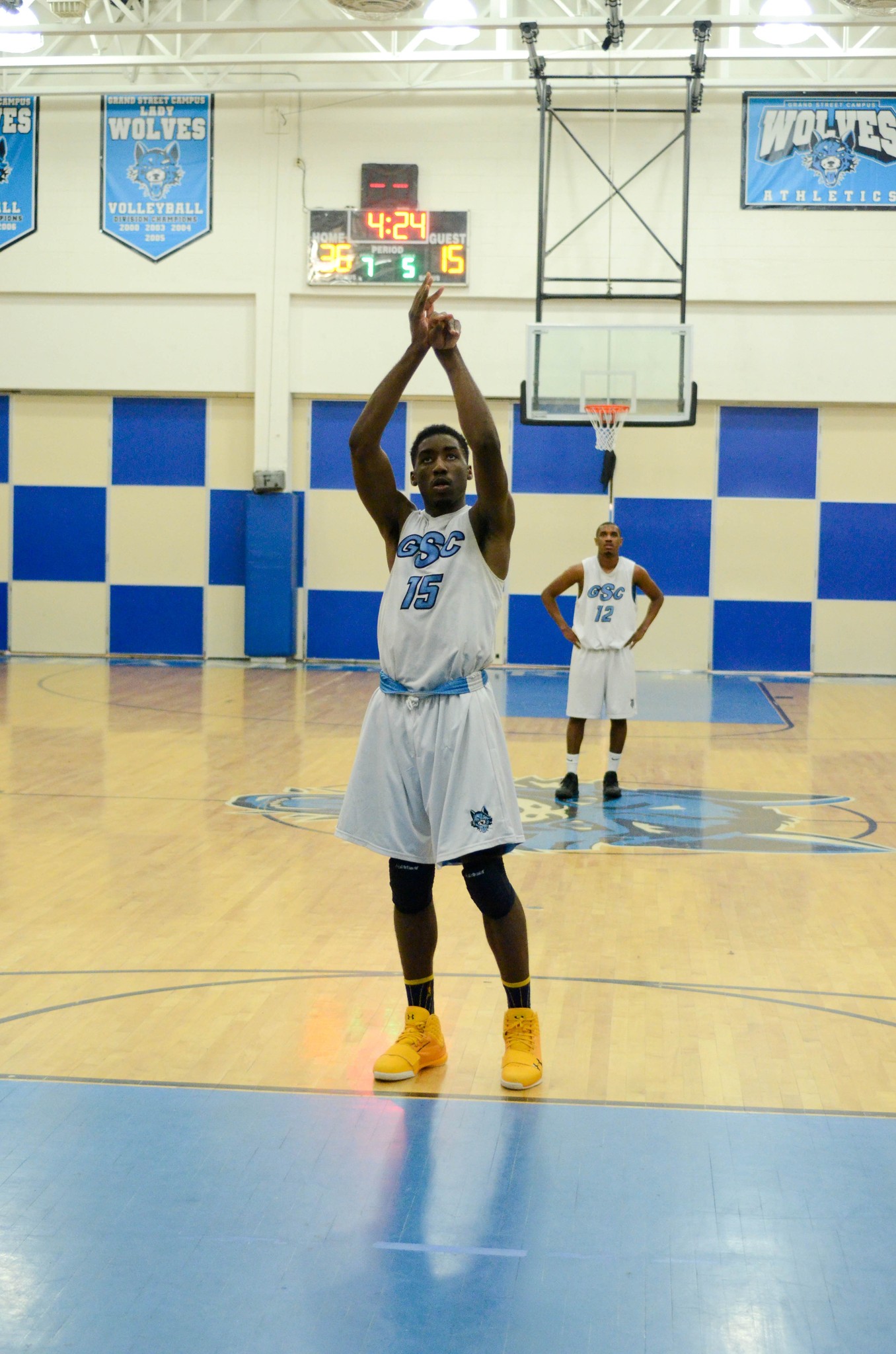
[[335, 274, 542, 1093], [541, 522, 664, 799]]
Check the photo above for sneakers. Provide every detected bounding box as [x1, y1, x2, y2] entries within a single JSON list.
[[373, 1006, 447, 1081], [602, 776, 622, 798], [501, 1008, 543, 1090], [555, 773, 579, 799]]
